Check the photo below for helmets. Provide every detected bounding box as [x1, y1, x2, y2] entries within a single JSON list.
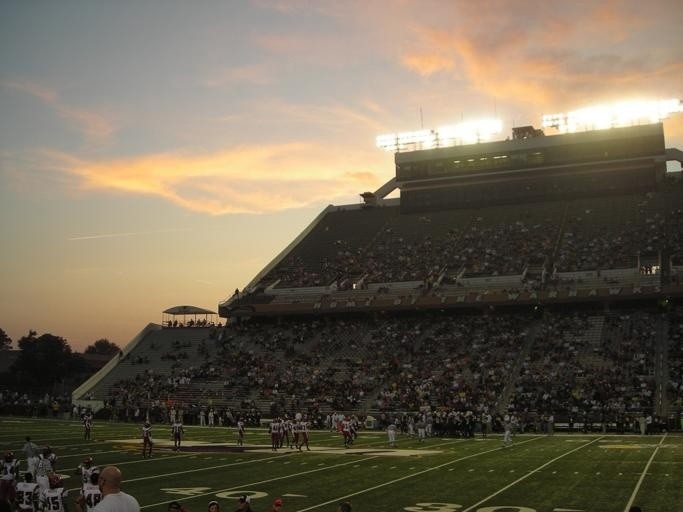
[[208, 501, 220, 510]]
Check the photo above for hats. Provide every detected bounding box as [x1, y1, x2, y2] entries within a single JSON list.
[[274, 498, 283, 507], [239, 496, 251, 503]]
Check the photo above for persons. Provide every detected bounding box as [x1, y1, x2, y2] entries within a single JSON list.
[[273, 499, 283, 512], [266, 174, 682, 301], [74, 456, 103, 510], [504, 421, 513, 444], [168, 502, 181, 512], [237, 419, 245, 444], [83, 313, 682, 437], [142, 423, 152, 458], [388, 423, 398, 446], [84, 415, 91, 440], [418, 421, 425, 442], [95, 466, 140, 512], [238, 495, 251, 512], [268, 418, 310, 451], [3, 390, 85, 420], [208, 500, 219, 512], [172, 418, 184, 450], [340, 502, 352, 512], [341, 416, 358, 448], [0, 436, 69, 512]]
